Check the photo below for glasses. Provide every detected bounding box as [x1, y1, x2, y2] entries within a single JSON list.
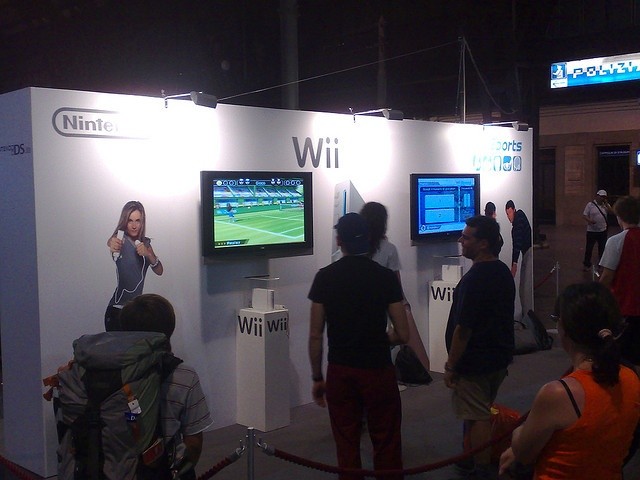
[[551, 310, 563, 324]]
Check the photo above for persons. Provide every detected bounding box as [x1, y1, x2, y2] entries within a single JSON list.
[[104, 200, 163, 331], [505, 200, 535, 319], [498, 283, 640, 480], [359, 201, 401, 280], [484, 202, 497, 219], [597, 196, 640, 369], [43, 293, 214, 480], [444, 215, 516, 466], [307, 212, 409, 480], [582, 189, 614, 266]]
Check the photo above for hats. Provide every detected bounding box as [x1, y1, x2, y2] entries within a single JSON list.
[[333, 213, 369, 239], [598, 190, 608, 197]]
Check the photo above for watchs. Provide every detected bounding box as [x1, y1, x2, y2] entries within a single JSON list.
[[445, 362, 452, 372]]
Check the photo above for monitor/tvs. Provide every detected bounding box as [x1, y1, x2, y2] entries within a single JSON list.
[[203, 171, 314, 257], [410, 174, 479, 238]]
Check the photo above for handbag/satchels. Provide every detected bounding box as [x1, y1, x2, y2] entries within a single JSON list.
[[514, 320, 539, 355], [394, 341, 434, 387], [592, 201, 610, 236]]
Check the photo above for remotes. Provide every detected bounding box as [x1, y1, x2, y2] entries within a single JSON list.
[[112, 230, 124, 257], [135, 239, 141, 245]]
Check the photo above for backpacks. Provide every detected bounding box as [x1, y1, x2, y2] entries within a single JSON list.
[[45, 326, 183, 480]]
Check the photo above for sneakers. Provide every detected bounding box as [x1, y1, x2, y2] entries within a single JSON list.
[[584, 265, 593, 272]]
